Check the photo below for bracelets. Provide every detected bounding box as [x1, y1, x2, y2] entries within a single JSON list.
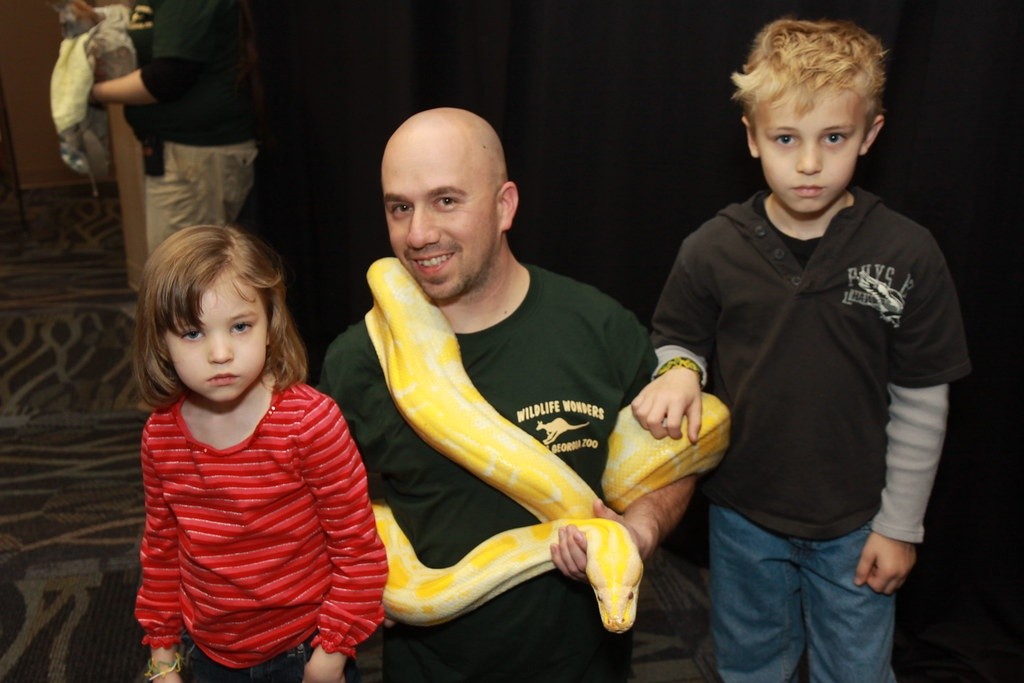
[[145, 651, 186, 680]]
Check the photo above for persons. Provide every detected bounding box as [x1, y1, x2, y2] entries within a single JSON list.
[[132, 223, 389, 683], [313, 108, 698, 682], [92, 0, 256, 411], [652, 19, 974, 683]]
